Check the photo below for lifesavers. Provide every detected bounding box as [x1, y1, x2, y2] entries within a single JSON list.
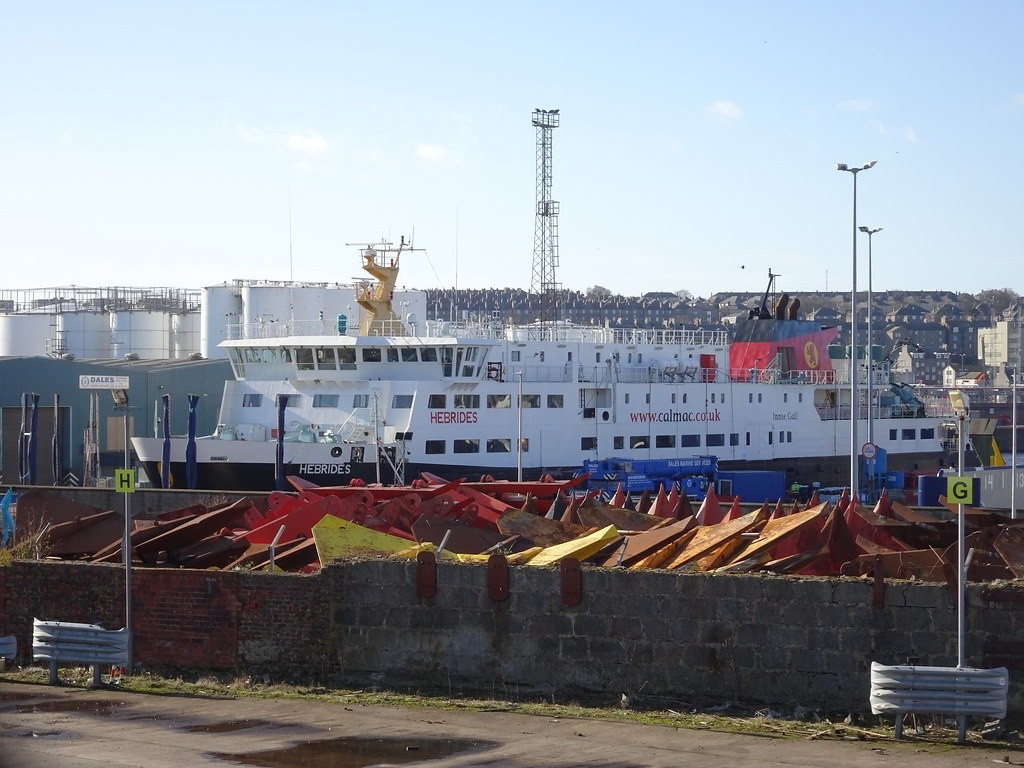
[[489, 367, 499, 378], [330, 446, 342, 457]]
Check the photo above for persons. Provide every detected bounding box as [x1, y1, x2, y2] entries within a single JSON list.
[[791, 481, 800, 502]]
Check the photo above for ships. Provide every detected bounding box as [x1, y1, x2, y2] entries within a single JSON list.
[[128, 233, 954, 494]]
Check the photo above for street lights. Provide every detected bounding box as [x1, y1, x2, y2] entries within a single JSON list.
[[948, 389, 971, 747], [1005, 364, 1017, 520], [858, 226, 885, 498], [835, 161, 879, 501], [111, 387, 133, 676], [959, 354, 966, 378], [515, 370, 525, 481]]
